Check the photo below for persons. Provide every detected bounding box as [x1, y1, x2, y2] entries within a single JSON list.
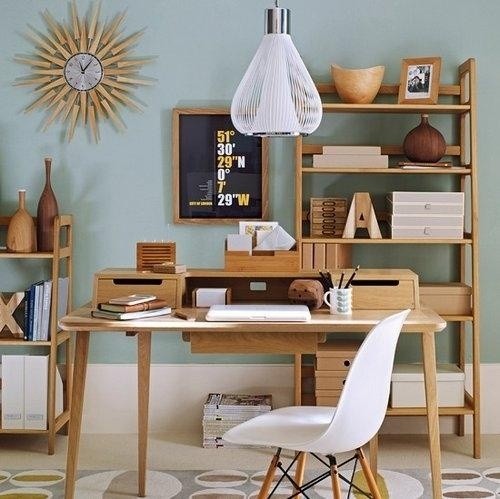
[[409, 68, 425, 92]]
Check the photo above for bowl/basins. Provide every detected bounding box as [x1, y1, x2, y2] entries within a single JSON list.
[[328, 64, 385, 104]]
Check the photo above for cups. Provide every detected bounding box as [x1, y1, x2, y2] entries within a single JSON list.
[[323, 285, 354, 317]]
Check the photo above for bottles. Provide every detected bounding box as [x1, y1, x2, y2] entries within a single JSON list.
[[37, 155, 59, 254], [5, 188, 39, 254], [402, 113, 445, 163]]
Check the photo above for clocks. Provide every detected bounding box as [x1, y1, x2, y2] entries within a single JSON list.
[[11, 0, 160, 146]]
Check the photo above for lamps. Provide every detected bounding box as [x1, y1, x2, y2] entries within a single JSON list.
[[231, 0, 322, 138]]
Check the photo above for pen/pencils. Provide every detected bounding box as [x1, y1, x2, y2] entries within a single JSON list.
[[317, 268, 334, 290], [338, 269, 345, 289], [344, 265, 359, 289]]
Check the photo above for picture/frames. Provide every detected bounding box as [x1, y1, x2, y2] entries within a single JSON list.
[[398, 56, 442, 105], [171, 106, 269, 227]]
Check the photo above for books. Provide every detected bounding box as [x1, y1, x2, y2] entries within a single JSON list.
[[93, 294, 171, 321], [202, 393, 273, 448], [311, 145, 390, 169], [2, 277, 69, 342]]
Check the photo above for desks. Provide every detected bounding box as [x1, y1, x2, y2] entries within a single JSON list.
[[57, 269, 446, 499]]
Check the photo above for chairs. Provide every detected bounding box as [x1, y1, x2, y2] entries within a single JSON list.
[[221, 308, 411, 499]]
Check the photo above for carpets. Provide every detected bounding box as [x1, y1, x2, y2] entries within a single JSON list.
[[0, 471, 500, 499]]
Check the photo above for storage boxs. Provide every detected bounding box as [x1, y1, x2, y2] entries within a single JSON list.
[[418, 282, 472, 316], [391, 362, 465, 408], [315, 340, 363, 407]]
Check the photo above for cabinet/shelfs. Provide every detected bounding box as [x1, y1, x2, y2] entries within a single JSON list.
[[0, 215, 72, 455], [295, 59, 481, 459]]
